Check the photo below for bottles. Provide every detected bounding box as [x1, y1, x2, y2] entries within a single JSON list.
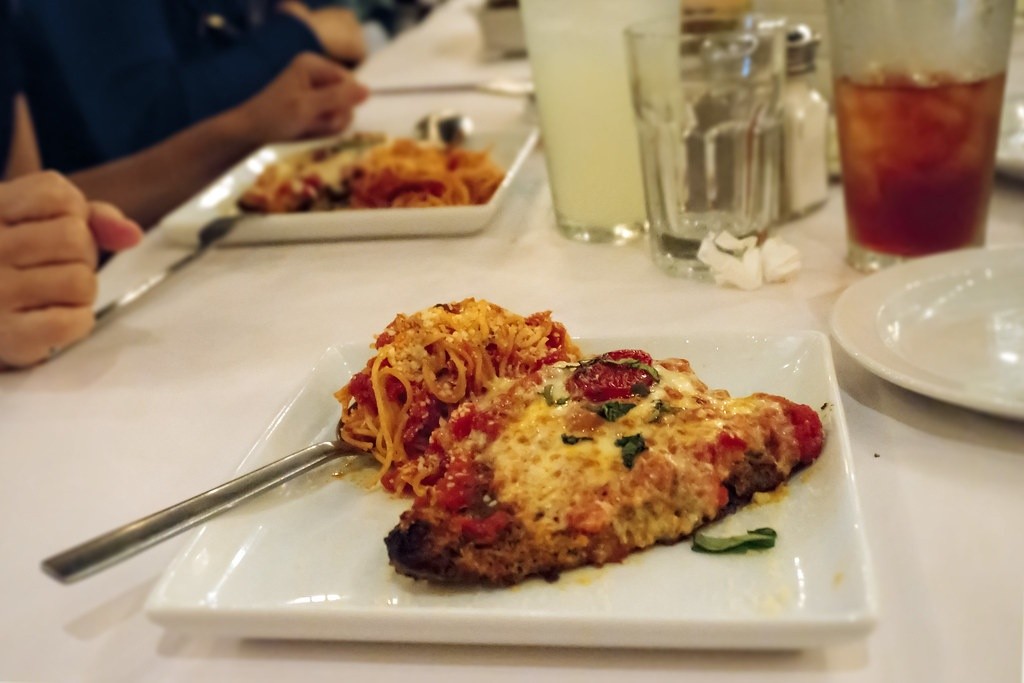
[[766, 23, 829, 221]]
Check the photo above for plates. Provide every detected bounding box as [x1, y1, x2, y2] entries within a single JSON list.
[[159, 126, 542, 246], [143, 330, 879, 650], [828, 245, 1023, 421]]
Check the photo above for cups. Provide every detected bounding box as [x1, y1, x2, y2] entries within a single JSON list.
[[824, 0, 1014, 274], [622, 13, 788, 277], [519, 0, 683, 245]]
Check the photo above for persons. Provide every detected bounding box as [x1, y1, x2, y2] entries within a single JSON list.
[[1, 0, 432, 369]]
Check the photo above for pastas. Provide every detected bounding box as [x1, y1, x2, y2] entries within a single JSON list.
[[356, 137, 509, 210], [340, 297, 575, 498]]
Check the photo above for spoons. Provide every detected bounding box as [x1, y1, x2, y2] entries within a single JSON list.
[[39, 401, 378, 585]]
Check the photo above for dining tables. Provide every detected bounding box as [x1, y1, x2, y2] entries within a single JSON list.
[[0, 0, 1024, 683]]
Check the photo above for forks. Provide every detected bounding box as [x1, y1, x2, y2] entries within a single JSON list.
[[93, 214, 261, 324]]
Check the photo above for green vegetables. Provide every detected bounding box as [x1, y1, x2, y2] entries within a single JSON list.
[[537, 353, 779, 552]]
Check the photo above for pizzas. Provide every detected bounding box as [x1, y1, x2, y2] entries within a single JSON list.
[[384, 358, 828, 584]]
